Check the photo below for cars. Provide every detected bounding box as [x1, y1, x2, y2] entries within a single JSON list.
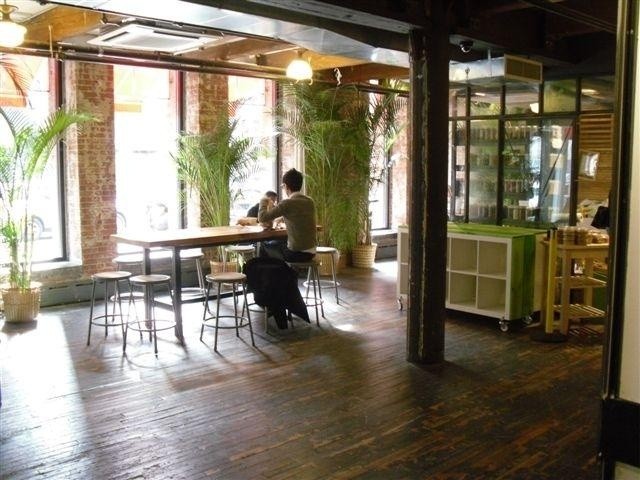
[[5, 190, 133, 242]]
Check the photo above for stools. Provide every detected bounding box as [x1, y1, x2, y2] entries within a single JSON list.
[[86, 246, 339, 355]]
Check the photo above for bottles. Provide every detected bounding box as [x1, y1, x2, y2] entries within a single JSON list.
[[457, 123, 532, 221]]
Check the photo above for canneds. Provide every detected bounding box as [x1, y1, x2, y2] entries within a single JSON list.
[[470, 128, 496, 139], [477, 204, 528, 220], [504, 180, 524, 193]]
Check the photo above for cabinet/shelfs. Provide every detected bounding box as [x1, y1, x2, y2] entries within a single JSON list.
[[455, 130, 567, 222], [540, 228, 610, 337]]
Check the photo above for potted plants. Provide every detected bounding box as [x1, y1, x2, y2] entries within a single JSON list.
[[263, 80, 376, 277], [335, 77, 409, 269], [168, 98, 260, 293], [0, 98, 104, 323]]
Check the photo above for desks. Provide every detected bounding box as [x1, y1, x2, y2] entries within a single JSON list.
[[109, 223, 323, 346]]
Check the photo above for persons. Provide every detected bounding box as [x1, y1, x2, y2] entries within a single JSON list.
[[258, 167, 319, 262], [245, 191, 278, 245]]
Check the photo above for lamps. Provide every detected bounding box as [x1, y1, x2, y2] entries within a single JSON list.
[[285, 51, 313, 80], [0, 0, 27, 47]]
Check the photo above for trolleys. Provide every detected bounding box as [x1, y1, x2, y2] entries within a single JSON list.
[[390, 216, 547, 332]]
